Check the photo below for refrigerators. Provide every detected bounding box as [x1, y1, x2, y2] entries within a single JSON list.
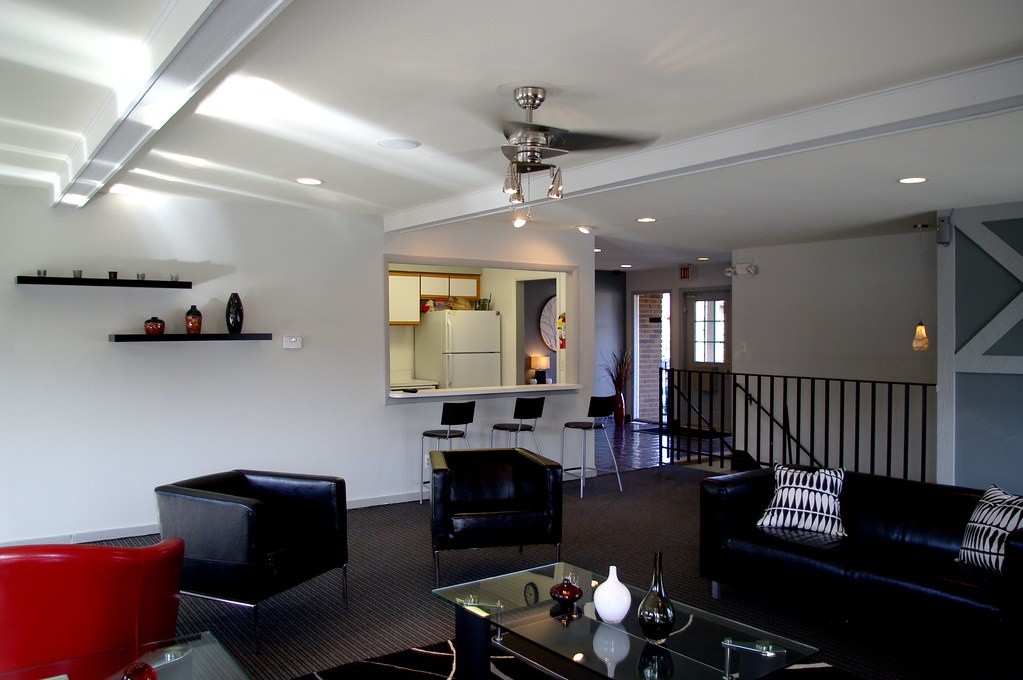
[[414, 310, 502, 388]]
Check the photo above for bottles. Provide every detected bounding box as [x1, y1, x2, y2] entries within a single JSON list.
[[550, 579, 583, 603], [145, 317, 165, 334], [186, 305, 202, 334], [550, 603, 582, 626], [638, 641, 673, 680], [637, 552, 675, 639], [593, 623, 630, 677], [226, 293, 243, 334], [593, 565, 631, 624]]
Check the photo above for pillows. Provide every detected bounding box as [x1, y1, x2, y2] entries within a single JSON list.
[[757, 461, 848, 538], [954, 482, 1022, 576]]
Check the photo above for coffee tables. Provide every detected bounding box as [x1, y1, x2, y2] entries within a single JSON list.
[[431, 562, 820, 680]]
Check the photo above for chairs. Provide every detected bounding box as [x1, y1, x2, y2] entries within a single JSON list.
[[153, 470, 348, 654], [428, 448, 564, 589], [0, 537, 187, 680]]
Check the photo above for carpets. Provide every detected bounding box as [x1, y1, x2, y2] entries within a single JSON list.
[[294, 601, 864, 680], [632, 425, 731, 442]]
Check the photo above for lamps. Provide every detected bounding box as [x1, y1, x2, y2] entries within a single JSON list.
[[531, 356, 551, 383], [723, 263, 758, 277], [911, 224, 929, 350], [578, 226, 592, 234], [500, 87, 569, 218], [513, 217, 527, 228]]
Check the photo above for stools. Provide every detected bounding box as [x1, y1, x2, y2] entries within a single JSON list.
[[561, 394, 624, 499], [420, 400, 476, 505], [490, 397, 546, 456]]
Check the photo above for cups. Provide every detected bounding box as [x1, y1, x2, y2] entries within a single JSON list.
[[109, 272, 117, 279], [171, 274, 179, 281], [37, 267, 46, 276], [73, 270, 82, 277], [137, 274, 145, 279]]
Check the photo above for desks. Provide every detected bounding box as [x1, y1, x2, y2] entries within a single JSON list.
[[0, 630, 253, 680]]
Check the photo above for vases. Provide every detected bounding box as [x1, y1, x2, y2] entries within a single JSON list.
[[226, 293, 244, 334], [615, 392, 624, 427], [637, 641, 673, 680], [594, 565, 632, 623], [592, 622, 631, 677], [549, 603, 583, 627], [186, 305, 203, 334], [550, 579, 583, 605], [637, 551, 676, 644], [144, 317, 165, 335]]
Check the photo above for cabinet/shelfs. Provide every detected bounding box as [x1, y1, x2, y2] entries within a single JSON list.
[[389, 271, 481, 325], [15, 276, 274, 342]]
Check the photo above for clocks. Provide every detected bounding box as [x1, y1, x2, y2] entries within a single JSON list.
[[525, 582, 539, 605]]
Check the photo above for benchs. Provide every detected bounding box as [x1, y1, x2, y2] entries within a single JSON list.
[[700, 464, 1023, 653]]
